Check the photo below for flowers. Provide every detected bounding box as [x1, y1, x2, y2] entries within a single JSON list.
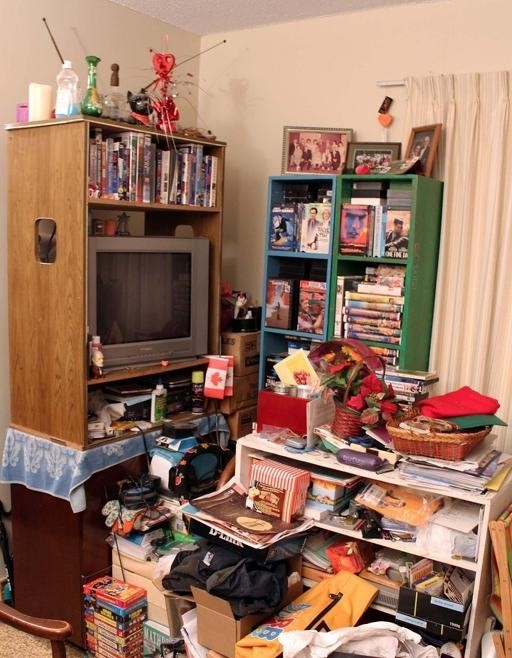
[[321, 355, 412, 431]]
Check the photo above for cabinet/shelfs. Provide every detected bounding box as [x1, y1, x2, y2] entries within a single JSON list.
[[257, 174, 337, 389], [327, 174, 444, 372], [234, 434, 512, 658], [8, 412, 224, 649], [6, 115, 227, 452]]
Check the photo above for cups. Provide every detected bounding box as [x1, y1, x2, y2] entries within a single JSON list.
[[26, 83, 56, 123]]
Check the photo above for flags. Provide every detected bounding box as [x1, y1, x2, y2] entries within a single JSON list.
[[224, 357, 235, 397], [203, 358, 229, 400]]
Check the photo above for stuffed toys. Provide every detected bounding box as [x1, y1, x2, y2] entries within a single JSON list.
[[125, 87, 153, 127]]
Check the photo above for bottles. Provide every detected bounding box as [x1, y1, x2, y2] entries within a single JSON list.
[[150, 378, 167, 423], [55, 59, 79, 118], [102, 86, 126, 121], [269, 382, 311, 399], [191, 372, 205, 415], [91, 336, 104, 378], [233, 291, 247, 319]]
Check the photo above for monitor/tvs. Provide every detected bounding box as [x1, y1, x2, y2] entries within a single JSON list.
[[88, 235, 212, 373]]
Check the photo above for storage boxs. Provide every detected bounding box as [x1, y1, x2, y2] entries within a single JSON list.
[[153, 554, 304, 658], [222, 332, 261, 441]]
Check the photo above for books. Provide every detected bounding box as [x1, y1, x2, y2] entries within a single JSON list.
[[89, 126, 219, 208], [333, 264, 406, 347], [367, 346, 440, 412], [264, 276, 327, 335], [340, 181, 412, 260], [270, 183, 332, 253]]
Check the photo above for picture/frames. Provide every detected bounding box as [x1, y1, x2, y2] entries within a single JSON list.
[[346, 142, 401, 174], [405, 123, 442, 176], [282, 126, 353, 176]]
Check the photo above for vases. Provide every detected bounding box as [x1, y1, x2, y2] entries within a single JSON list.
[[330, 398, 364, 441]]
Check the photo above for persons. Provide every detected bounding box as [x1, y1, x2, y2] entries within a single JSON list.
[[408, 136, 431, 172], [92, 350, 105, 379], [288, 138, 344, 172], [356, 152, 391, 168]]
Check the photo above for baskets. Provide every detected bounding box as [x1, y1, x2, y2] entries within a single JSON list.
[[331, 356, 385, 438], [385, 407, 494, 461]]
